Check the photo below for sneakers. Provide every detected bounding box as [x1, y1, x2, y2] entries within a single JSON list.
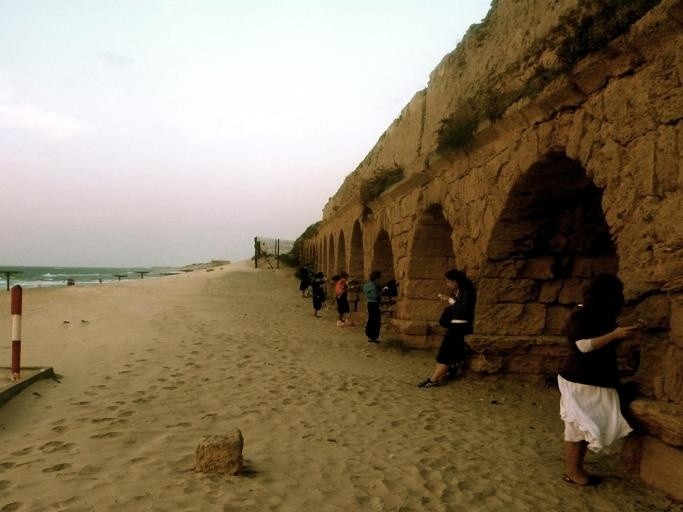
[[367, 340, 381, 345], [417, 377, 448, 388]]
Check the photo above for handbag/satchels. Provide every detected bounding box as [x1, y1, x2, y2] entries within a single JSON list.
[[439, 305, 454, 328]]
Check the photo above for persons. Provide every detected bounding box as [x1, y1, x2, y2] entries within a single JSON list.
[[311, 271, 327, 317], [556, 272, 649, 485], [298, 265, 311, 298], [364, 270, 388, 342], [334, 272, 351, 327], [417, 269, 476, 388]]
